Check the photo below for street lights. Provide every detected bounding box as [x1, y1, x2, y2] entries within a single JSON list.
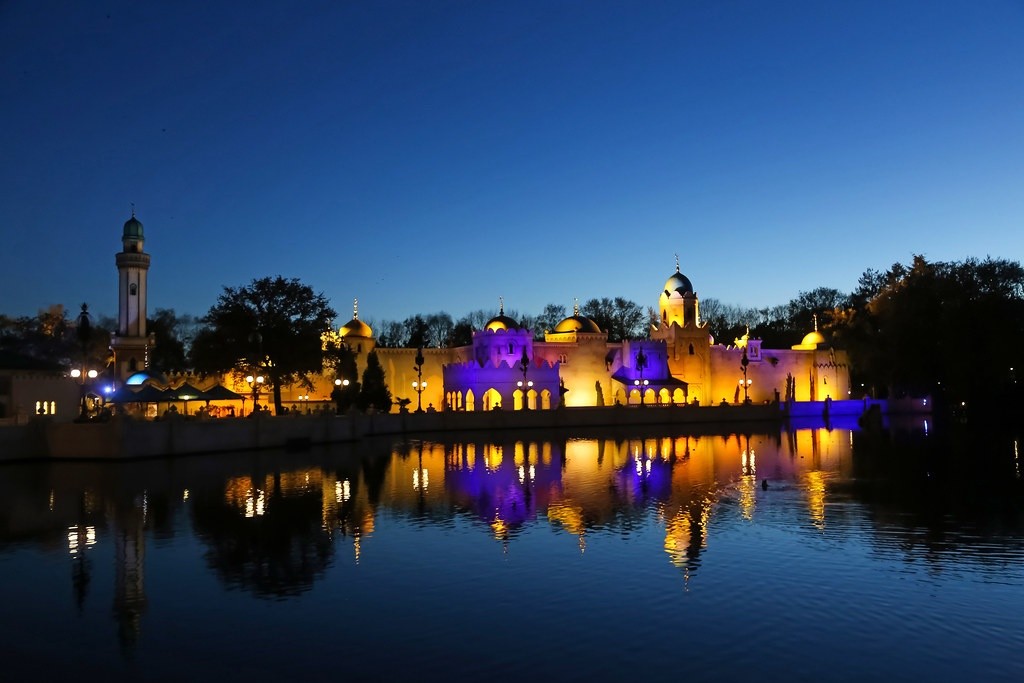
[[334, 475, 351, 538], [518, 441, 535, 514], [739, 348, 752, 405], [741, 433, 756, 477], [66, 490, 98, 569], [334, 336, 350, 414], [412, 442, 429, 528], [634, 346, 649, 408], [104, 348, 117, 416], [516, 346, 533, 410], [305, 394, 309, 409], [71, 300, 98, 423], [246, 376, 264, 412], [412, 347, 427, 413], [244, 453, 266, 518], [298, 394, 303, 408], [636, 438, 652, 496]]
